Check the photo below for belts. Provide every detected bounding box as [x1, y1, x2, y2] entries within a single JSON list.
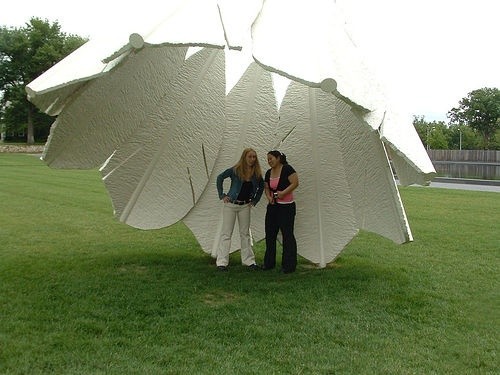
[[229, 200, 250, 205]]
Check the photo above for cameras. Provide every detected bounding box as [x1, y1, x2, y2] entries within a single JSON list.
[[274, 193, 278, 198]]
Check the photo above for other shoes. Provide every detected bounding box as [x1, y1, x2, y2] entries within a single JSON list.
[[218, 266, 228, 272], [261, 264, 274, 270], [250, 264, 259, 271], [279, 267, 290, 273]]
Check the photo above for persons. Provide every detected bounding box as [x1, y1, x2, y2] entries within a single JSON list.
[[216, 148, 264, 270], [260, 150, 299, 273]]
